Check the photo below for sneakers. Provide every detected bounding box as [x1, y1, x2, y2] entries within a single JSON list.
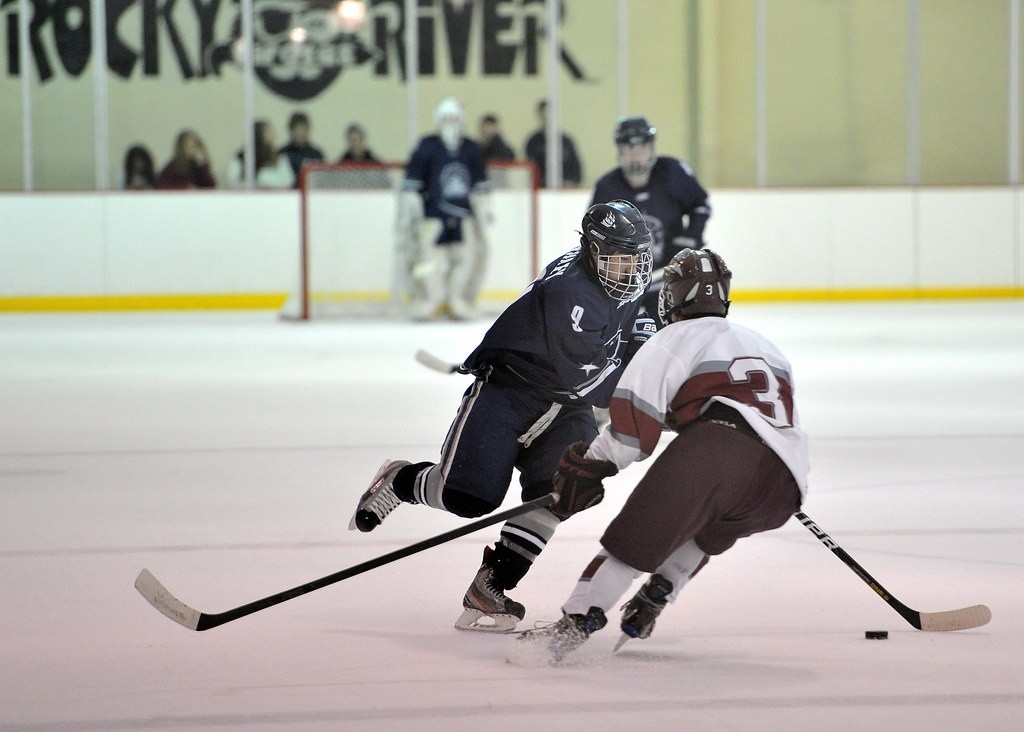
[[349, 460, 413, 533], [454, 545, 526, 631], [508, 605, 608, 671], [613, 574, 674, 651]]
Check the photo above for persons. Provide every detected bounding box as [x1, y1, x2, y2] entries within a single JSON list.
[[279, 112, 322, 189], [332, 126, 389, 187], [226, 114, 296, 188], [525, 102, 581, 188], [516, 248, 808, 658], [350, 200, 656, 630], [392, 101, 492, 320], [593, 118, 711, 424], [478, 117, 515, 162], [124, 131, 215, 189]]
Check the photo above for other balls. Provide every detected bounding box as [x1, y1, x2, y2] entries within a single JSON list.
[[865, 630, 889, 640]]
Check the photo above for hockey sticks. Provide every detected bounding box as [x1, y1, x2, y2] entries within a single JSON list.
[[795, 505, 995, 636], [413, 274, 665, 375], [133, 491, 560, 634]]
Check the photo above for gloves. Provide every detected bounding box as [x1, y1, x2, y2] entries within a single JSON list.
[[549, 442, 618, 518]]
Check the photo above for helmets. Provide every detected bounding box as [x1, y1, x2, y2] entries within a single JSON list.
[[437, 100, 464, 142], [577, 200, 655, 303], [658, 248, 733, 326], [615, 118, 658, 188]]
